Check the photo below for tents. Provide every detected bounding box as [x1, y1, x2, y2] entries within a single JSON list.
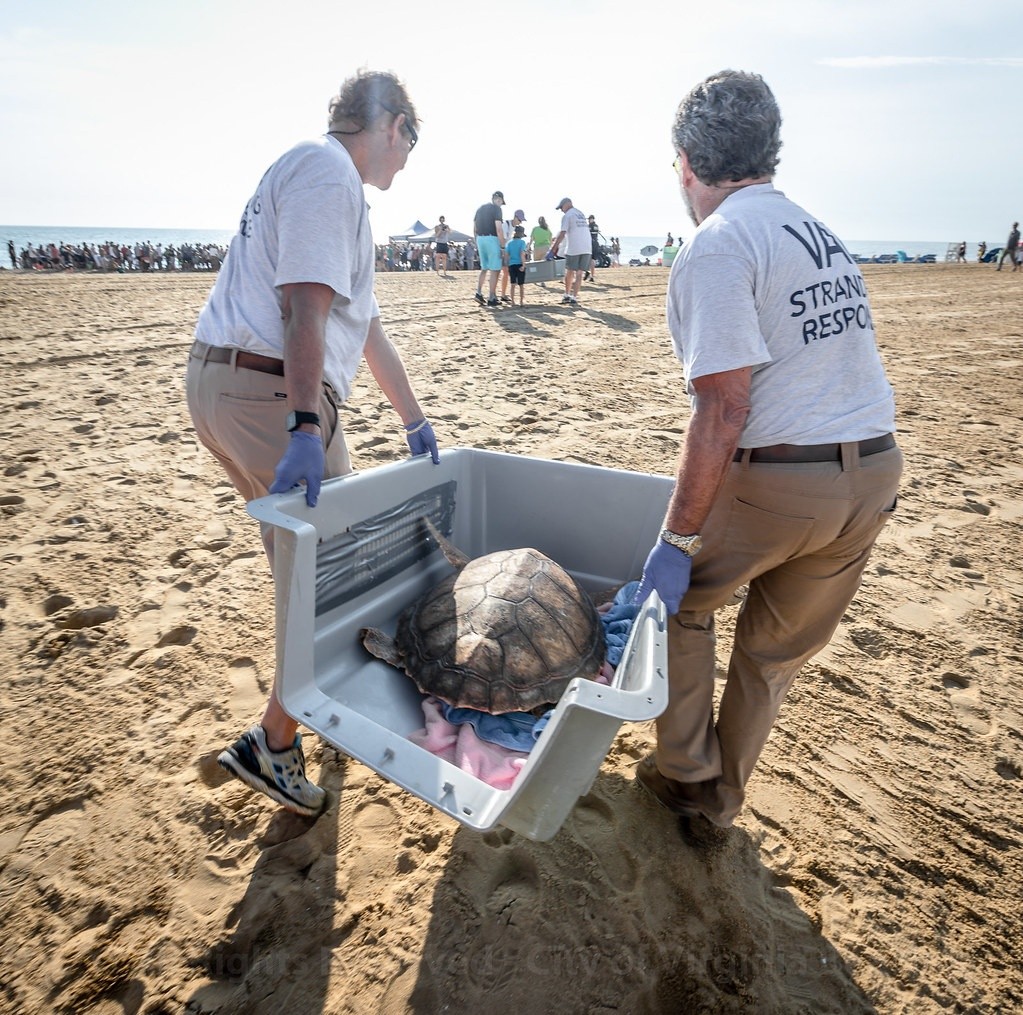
[[389, 220, 474, 243]]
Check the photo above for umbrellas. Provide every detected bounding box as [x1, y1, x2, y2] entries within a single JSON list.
[[640, 245, 658, 256]]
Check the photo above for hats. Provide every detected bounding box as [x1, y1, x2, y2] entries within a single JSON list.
[[515, 210, 527, 222], [556, 197, 572, 210], [587, 215, 595, 219], [493, 192, 506, 206]]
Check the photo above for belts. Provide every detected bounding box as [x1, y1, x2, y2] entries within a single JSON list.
[[189, 341, 284, 377], [730, 433, 896, 463]]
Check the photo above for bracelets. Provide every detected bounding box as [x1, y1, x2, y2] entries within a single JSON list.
[[501, 247, 505, 249], [407, 420, 427, 434]]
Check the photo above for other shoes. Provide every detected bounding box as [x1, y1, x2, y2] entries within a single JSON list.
[[562, 296, 571, 303], [488, 299, 502, 305], [570, 300, 578, 304], [584, 272, 590, 280], [636, 750, 727, 847], [501, 296, 512, 301], [474, 293, 488, 305], [589, 278, 594, 281]]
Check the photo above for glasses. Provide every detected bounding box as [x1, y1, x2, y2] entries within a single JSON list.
[[380, 100, 419, 153], [672, 156, 684, 174]]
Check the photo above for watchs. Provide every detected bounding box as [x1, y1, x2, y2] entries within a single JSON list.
[[658, 527, 704, 558], [285, 410, 321, 433]]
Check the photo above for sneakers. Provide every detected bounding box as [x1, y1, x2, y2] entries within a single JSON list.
[[218, 728, 328, 812]]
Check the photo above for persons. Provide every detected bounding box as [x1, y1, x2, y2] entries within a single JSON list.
[[632, 69, 902, 842], [8, 240, 230, 272], [977, 242, 986, 262], [375, 238, 480, 273], [958, 241, 966, 263], [472, 191, 527, 306], [996, 221, 1020, 272], [666, 232, 684, 246], [186, 73, 441, 811], [528, 197, 601, 304], [434, 216, 450, 276], [610, 237, 620, 267], [645, 258, 650, 265]]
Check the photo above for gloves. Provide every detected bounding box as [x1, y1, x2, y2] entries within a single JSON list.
[[633, 539, 694, 614], [545, 251, 555, 260], [267, 431, 324, 508], [403, 418, 442, 465]]
